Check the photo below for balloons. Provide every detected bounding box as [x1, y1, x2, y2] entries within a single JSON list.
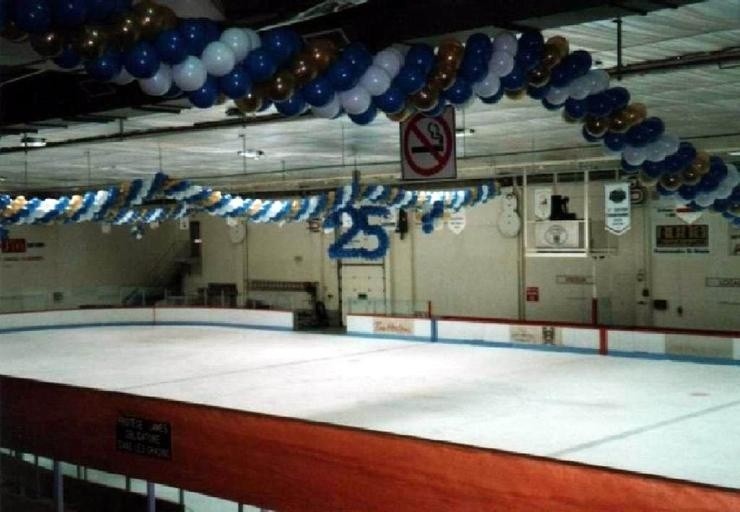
[[1, 172, 502, 259], [1, 2, 568, 124], [561, 50, 739, 227]]
